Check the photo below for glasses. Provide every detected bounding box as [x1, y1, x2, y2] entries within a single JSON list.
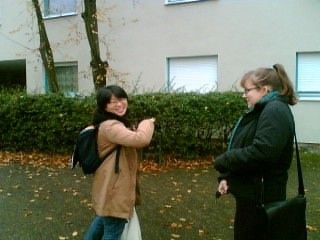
[[245, 87, 259, 94]]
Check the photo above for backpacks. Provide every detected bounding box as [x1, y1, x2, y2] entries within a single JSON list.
[[71, 116, 128, 176]]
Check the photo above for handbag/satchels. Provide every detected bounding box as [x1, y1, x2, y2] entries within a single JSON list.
[[256, 195, 307, 240]]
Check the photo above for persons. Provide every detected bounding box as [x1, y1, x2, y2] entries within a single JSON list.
[[214, 63, 298, 240], [82, 85, 156, 240]]
